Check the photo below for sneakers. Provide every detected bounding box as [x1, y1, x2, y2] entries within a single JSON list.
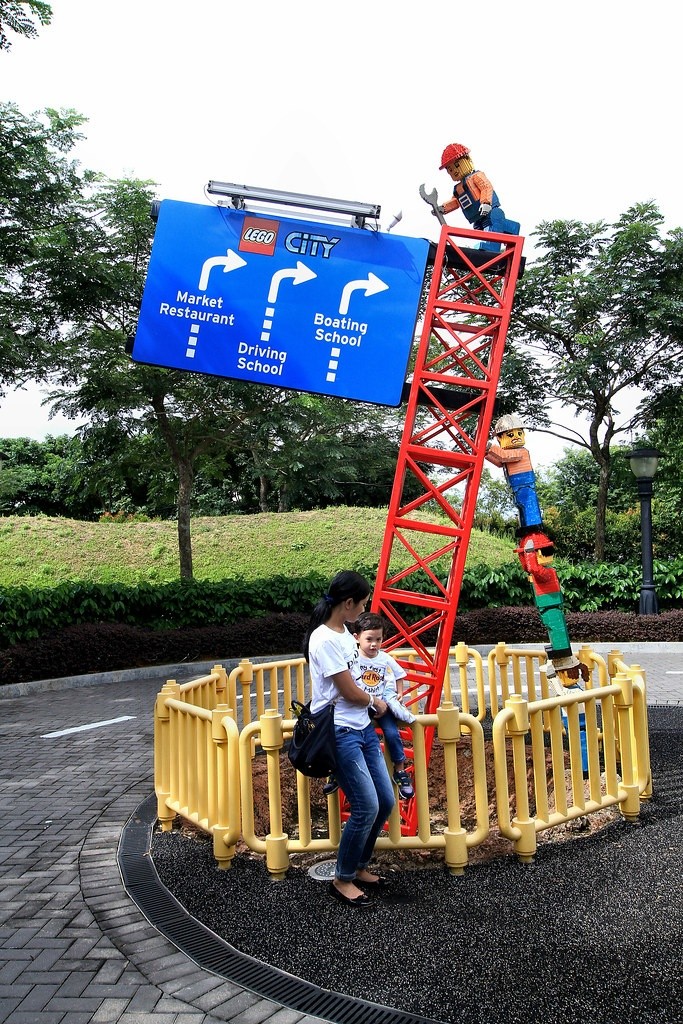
[[321, 774, 338, 795], [392, 769, 417, 798]]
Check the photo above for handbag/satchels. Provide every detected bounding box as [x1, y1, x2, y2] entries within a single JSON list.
[[287, 699, 338, 778]]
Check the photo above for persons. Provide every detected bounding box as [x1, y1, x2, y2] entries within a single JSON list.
[[538, 656, 600, 780], [484, 414, 544, 536], [301, 570, 398, 909], [430, 143, 521, 250], [323, 612, 417, 801], [512, 531, 573, 660]]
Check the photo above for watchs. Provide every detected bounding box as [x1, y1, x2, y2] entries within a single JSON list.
[[363, 693, 375, 709]]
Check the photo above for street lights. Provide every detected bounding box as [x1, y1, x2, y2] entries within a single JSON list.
[[623, 441, 670, 615]]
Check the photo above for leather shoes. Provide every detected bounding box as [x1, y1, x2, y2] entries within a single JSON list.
[[328, 881, 375, 907], [352, 872, 391, 889]]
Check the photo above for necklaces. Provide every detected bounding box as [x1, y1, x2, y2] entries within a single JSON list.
[[323, 622, 345, 634]]
